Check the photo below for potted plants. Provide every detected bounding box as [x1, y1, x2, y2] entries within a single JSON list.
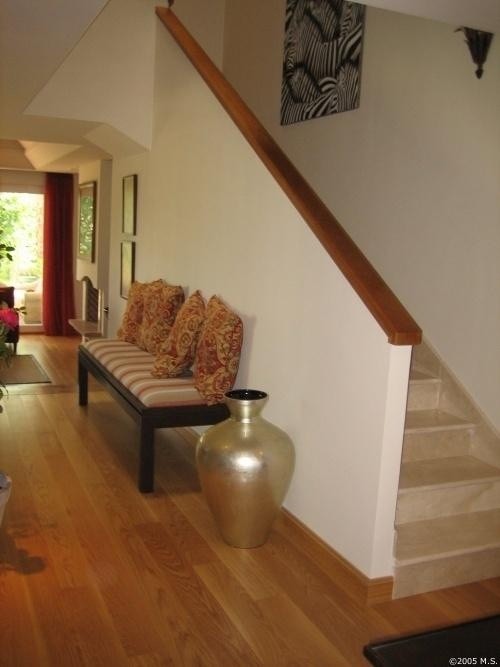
[[0, 355, 16, 556]]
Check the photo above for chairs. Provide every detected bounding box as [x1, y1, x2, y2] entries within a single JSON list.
[[1, 281, 21, 353]]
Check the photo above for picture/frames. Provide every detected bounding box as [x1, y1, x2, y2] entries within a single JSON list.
[[119, 172, 140, 303]]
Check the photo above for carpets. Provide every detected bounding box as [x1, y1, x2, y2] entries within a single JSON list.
[[360, 611, 498, 665], [0, 352, 51, 385]]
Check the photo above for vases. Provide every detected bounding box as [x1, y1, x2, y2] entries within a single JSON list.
[[191, 385, 302, 551]]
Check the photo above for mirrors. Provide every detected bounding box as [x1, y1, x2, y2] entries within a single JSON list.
[[76, 179, 96, 262]]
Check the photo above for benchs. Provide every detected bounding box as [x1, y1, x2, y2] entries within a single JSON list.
[[78, 333, 237, 493]]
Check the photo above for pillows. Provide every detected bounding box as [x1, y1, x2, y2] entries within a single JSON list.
[[117, 279, 244, 405]]
